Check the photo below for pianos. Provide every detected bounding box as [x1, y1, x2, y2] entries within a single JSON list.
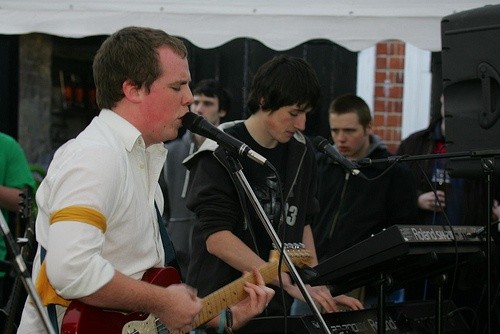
[[240, 299, 463, 334], [302, 223, 500, 295]]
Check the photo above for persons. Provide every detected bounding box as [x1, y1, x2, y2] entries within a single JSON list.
[[17, 26, 275, 334], [291, 94, 401, 316], [389, 92, 500, 307], [183, 54, 363, 317], [0, 132, 35, 302], [152, 79, 229, 285]]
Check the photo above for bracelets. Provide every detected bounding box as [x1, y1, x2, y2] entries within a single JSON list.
[[217, 308, 233, 334]]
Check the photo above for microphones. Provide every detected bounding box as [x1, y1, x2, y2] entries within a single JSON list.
[[313, 135, 363, 177], [181, 111, 267, 166]]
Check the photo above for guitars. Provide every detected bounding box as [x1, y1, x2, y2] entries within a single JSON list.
[[60, 242, 315, 334], [0, 184, 36, 334]]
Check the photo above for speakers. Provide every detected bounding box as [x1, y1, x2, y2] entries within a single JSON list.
[[440, 5, 500, 178]]
